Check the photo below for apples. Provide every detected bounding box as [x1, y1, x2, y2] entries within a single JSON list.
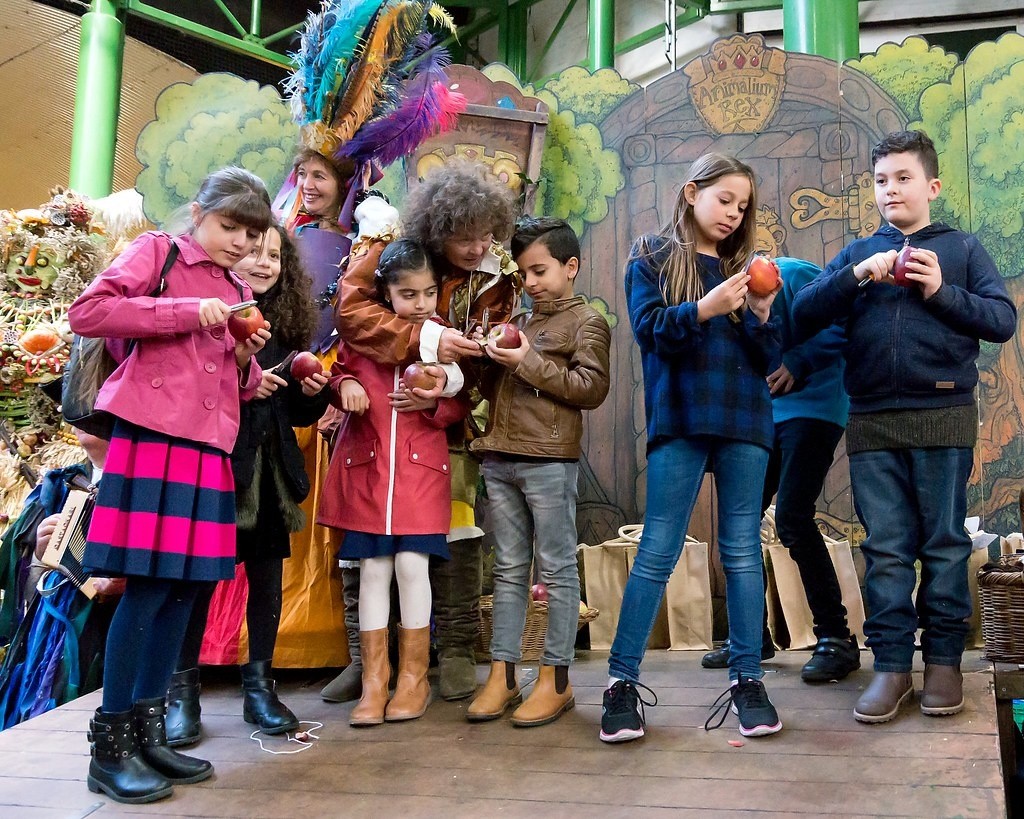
[[893, 246, 923, 288], [531, 584, 548, 601], [290, 352, 322, 382], [579, 600, 588, 612], [403, 363, 436, 391], [227, 305, 264, 343], [488, 323, 521, 349], [740, 257, 778, 297]]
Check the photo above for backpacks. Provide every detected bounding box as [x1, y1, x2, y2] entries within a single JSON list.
[[62, 237, 243, 442]]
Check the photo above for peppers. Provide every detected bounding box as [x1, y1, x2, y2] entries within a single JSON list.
[[17, 277, 42, 285]]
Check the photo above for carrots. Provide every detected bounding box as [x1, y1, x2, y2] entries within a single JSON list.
[[24, 242, 40, 266]]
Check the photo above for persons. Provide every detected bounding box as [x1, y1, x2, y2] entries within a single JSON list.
[[466, 216, 611, 730], [790, 130, 1017, 723], [0, 425, 126, 733], [701, 257, 860, 683], [321, 166, 524, 703], [165, 215, 331, 749], [198, 147, 367, 669], [599, 151, 784, 743], [67, 168, 272, 804], [327, 239, 473, 725]]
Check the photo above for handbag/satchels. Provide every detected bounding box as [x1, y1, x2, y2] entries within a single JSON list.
[[758, 505, 871, 652], [576, 524, 715, 652]]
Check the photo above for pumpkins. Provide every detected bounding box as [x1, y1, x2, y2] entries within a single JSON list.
[[18, 327, 58, 354]]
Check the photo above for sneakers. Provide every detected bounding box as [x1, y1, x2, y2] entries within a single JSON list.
[[704, 629, 775, 669], [802, 634, 863, 682], [599, 679, 658, 743], [705, 671, 783, 737]]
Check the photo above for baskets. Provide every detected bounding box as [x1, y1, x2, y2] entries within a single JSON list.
[[976, 563, 1024, 663], [478, 540, 600, 661]]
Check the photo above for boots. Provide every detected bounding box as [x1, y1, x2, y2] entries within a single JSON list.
[[385, 622, 432, 720], [240, 657, 299, 735], [511, 664, 576, 726], [164, 667, 202, 746], [428, 527, 485, 701], [349, 627, 389, 725], [132, 696, 213, 783], [320, 560, 395, 703], [87, 702, 173, 804], [466, 661, 522, 720]]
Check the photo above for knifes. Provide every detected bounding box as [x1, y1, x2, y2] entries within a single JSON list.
[[743, 250, 757, 276], [858, 245, 909, 287], [221, 300, 258, 314], [482, 306, 489, 338], [271, 350, 298, 375], [461, 319, 477, 338]]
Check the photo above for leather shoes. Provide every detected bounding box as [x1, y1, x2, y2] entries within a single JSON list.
[[921, 661, 964, 714], [852, 669, 915, 723]]
